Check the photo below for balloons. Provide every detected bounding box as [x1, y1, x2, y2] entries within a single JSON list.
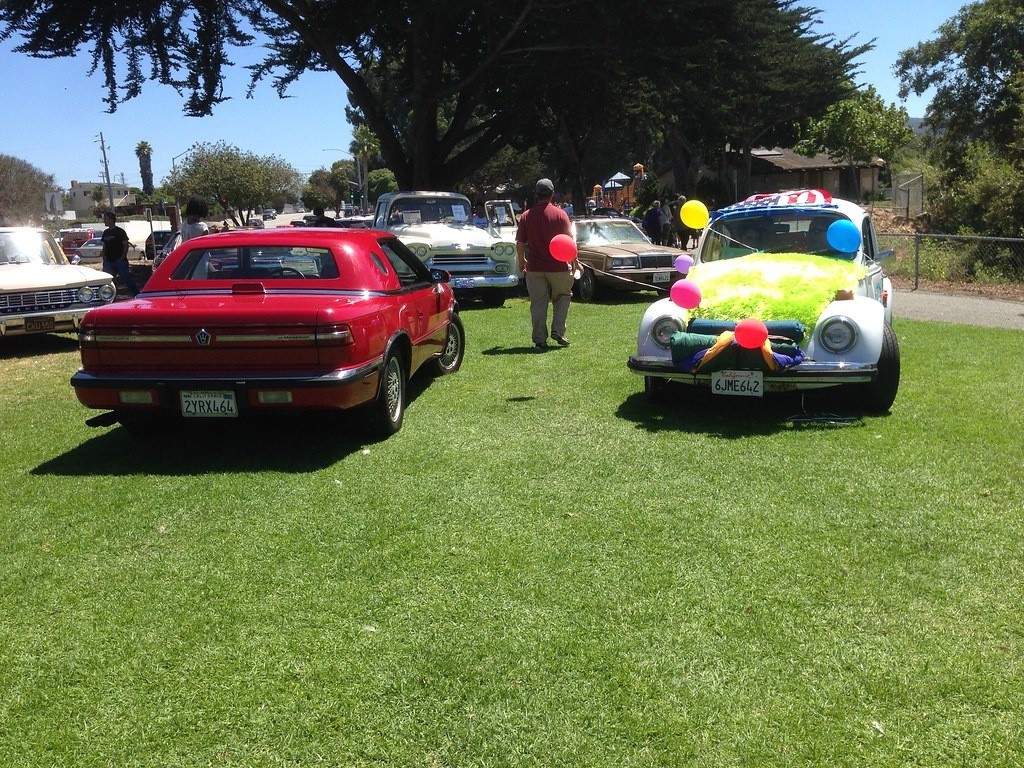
[[681, 199, 709, 229], [735, 318, 767, 349], [675, 254, 694, 275], [549, 234, 577, 263], [827, 218, 860, 252], [670, 279, 701, 308]]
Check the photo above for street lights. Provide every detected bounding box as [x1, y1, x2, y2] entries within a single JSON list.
[[322, 148, 363, 215], [93, 131, 118, 225]]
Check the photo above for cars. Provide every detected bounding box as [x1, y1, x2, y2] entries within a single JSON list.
[[333, 218, 373, 231], [276, 224, 295, 229], [372, 190, 521, 309], [70, 227, 466, 444], [564, 218, 697, 302], [152, 229, 283, 281], [561, 203, 637, 224], [263, 210, 276, 221], [145, 230, 174, 261], [74, 237, 146, 264], [297, 208, 305, 213], [291, 220, 311, 229], [248, 219, 264, 229], [627, 188, 901, 417], [344, 206, 361, 218], [303, 215, 320, 227], [271, 208, 278, 216], [0, 225, 117, 347]]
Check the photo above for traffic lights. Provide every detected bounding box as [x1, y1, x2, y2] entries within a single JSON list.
[[145, 209, 152, 225], [351, 189, 354, 199], [361, 191, 364, 198]]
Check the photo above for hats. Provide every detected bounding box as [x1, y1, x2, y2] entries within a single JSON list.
[[536, 178, 554, 194]]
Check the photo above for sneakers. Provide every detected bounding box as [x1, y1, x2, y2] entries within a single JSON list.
[[550, 331, 570, 346], [534, 343, 548, 349]]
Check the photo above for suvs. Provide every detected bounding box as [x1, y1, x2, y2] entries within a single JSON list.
[[62, 229, 108, 261], [43, 229, 89, 256]]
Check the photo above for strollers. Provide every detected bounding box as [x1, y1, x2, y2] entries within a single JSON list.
[[666, 223, 683, 250]]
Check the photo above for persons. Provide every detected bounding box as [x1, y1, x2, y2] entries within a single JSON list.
[[181, 195, 211, 260], [101, 211, 140, 297], [643, 194, 700, 250], [517, 178, 584, 349], [474, 203, 494, 228], [599, 197, 604, 207], [554, 199, 597, 208], [313, 205, 336, 228]]
